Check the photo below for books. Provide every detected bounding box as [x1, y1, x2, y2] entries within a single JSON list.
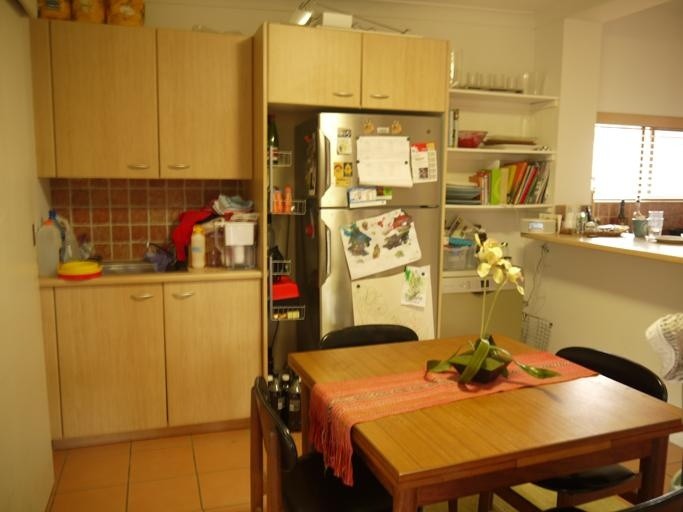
[[446, 156, 550, 205]]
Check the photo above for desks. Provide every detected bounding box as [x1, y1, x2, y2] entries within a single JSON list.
[[288, 333, 682, 511]]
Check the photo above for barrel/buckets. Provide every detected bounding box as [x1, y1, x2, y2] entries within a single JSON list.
[[35, 219, 62, 278]]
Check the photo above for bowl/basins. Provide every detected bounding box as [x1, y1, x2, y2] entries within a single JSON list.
[[458, 130, 490, 147]]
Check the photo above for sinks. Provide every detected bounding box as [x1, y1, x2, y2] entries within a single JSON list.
[[98, 259, 158, 273]]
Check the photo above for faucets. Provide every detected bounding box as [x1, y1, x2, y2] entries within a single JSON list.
[[143, 239, 177, 265]]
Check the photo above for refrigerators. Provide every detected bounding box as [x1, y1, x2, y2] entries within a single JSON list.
[[294, 112, 442, 351]]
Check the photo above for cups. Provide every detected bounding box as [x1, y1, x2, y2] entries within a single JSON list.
[[632, 219, 648, 237], [454, 71, 545, 94]]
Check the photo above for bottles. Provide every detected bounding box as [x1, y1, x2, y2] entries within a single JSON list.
[[633, 200, 642, 218], [191, 227, 205, 268], [269, 114, 279, 162], [618, 200, 627, 224], [267, 373, 303, 433], [577, 212, 588, 234], [273, 183, 292, 213], [648, 211, 664, 242], [36, 209, 66, 278]]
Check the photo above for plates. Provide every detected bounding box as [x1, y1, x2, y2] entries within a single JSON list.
[[655, 235, 683, 245], [594, 224, 630, 236]]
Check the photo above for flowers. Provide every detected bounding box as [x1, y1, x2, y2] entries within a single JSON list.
[[423, 233, 564, 384]]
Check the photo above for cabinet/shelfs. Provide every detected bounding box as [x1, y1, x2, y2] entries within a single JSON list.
[[266, 256, 306, 322], [266, 22, 446, 114], [268, 144, 307, 217], [29, 18, 253, 181], [39, 278, 263, 450], [445, 88, 559, 209]]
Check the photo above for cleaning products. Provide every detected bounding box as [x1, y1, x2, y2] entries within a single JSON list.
[[58, 216, 82, 262]]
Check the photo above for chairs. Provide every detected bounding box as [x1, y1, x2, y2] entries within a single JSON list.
[[319, 325, 418, 346], [251, 376, 423, 511], [536, 488, 682, 510], [475, 345, 668, 511]]
